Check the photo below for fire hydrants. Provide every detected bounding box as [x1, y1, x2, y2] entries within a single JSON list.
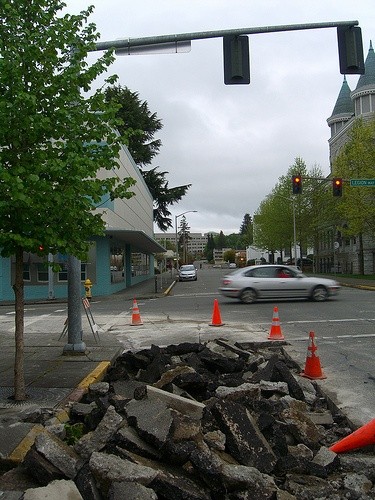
[[84, 278, 93, 302]]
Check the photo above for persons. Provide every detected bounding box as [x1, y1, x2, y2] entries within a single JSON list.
[[168, 260, 184, 272], [277, 268, 288, 278]]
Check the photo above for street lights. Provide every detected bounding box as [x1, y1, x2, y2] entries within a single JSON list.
[[174, 210, 198, 271]]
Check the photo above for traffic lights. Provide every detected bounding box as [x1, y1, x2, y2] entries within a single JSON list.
[[332, 177, 344, 197], [291, 174, 302, 195]]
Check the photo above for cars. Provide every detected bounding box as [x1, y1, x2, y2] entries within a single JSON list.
[[177, 265, 198, 281], [229, 263, 237, 269], [221, 264, 340, 305]]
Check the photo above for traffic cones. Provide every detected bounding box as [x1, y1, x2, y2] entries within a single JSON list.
[[130, 299, 145, 326], [330, 418, 375, 453], [207, 298, 226, 327], [297, 330, 328, 380], [266, 306, 284, 340]]
